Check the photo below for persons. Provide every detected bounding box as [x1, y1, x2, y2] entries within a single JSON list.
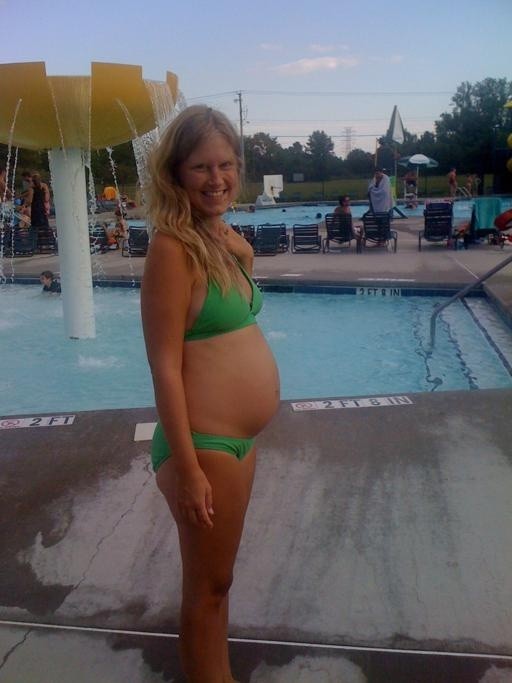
[[248, 205, 256, 213], [464, 173, 471, 193], [404, 191, 419, 208], [333, 195, 360, 243], [446, 167, 456, 199], [367, 165, 393, 223], [137, 101, 283, 683], [400, 168, 417, 188], [36, 269, 61, 293], [1, 169, 53, 251], [92, 189, 135, 250]]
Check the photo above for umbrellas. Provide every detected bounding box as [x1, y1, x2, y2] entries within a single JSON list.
[[399, 152, 439, 199]]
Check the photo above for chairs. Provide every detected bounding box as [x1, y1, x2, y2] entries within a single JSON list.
[[89, 222, 120, 254], [322, 213, 363, 255], [121, 225, 149, 257], [231, 223, 290, 256], [291, 224, 322, 253], [416, 196, 454, 250], [1, 207, 58, 258], [469, 194, 503, 245], [360, 210, 397, 253]]
